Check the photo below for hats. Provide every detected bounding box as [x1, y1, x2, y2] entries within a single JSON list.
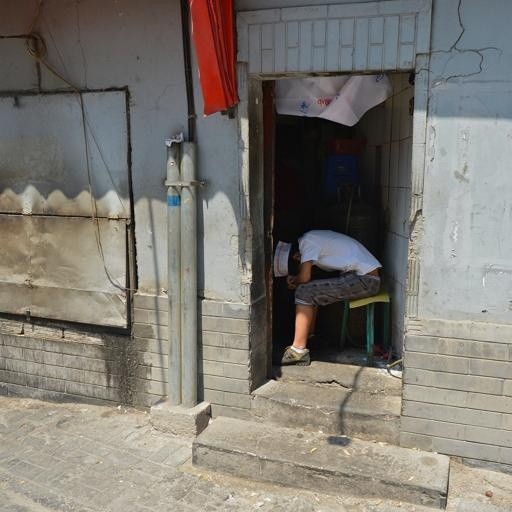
[[273, 241, 292, 277]]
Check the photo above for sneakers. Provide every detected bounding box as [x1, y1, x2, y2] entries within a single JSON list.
[[273, 346, 311, 366]]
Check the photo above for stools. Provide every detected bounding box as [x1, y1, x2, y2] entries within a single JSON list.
[[337, 289, 392, 352]]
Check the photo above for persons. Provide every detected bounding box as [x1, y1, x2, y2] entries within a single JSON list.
[[272, 230, 383, 366]]
